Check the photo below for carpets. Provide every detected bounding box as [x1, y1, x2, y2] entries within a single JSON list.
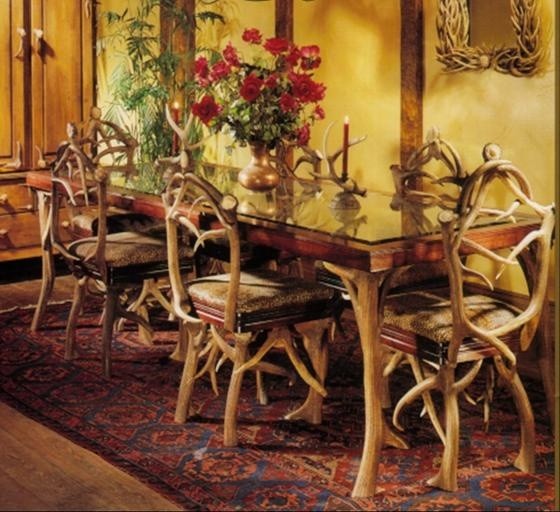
[[0, 294, 560, 512]]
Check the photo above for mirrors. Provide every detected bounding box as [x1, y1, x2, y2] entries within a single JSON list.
[[433, 0, 545, 81]]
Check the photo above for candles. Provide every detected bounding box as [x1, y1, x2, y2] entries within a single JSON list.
[[342, 115, 348, 179]]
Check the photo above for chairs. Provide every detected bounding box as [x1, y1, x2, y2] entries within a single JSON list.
[[389, 127, 468, 210], [282, 138, 324, 180], [160, 148, 347, 447], [55, 109, 140, 170], [29, 122, 209, 375], [377, 143, 556, 494]]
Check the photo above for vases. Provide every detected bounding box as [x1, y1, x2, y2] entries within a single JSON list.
[[236, 142, 279, 192]]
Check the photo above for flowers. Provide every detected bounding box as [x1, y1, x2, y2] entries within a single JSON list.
[[189, 29, 328, 150]]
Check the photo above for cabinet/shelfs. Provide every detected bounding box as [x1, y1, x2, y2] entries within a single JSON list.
[[0, 1, 98, 266]]
